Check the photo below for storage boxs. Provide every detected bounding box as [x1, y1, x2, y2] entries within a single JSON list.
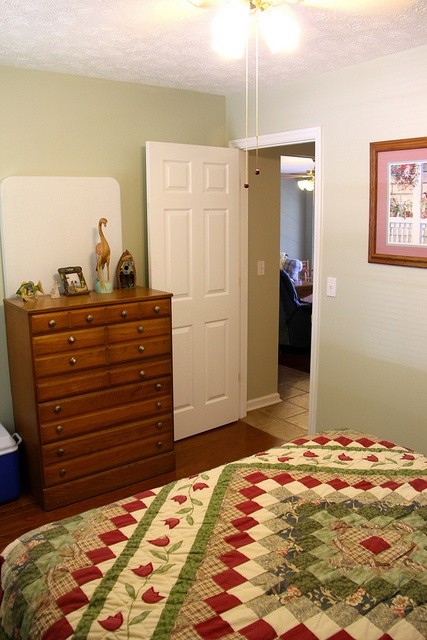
[[0, 423, 22, 504]]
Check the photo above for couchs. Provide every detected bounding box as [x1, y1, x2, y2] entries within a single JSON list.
[[279, 267, 312, 374]]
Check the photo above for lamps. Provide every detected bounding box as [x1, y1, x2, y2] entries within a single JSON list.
[[297, 169, 315, 191]]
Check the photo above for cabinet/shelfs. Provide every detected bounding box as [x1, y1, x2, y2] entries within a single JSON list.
[[3, 284, 176, 512]]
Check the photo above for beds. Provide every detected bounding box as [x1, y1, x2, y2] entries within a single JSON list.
[[0, 428, 426, 639]]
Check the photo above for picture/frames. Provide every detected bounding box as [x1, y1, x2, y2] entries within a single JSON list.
[[58, 267, 90, 296], [368, 137, 427, 270]]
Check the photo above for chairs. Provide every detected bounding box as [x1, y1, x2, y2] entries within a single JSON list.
[[298, 260, 313, 281]]
